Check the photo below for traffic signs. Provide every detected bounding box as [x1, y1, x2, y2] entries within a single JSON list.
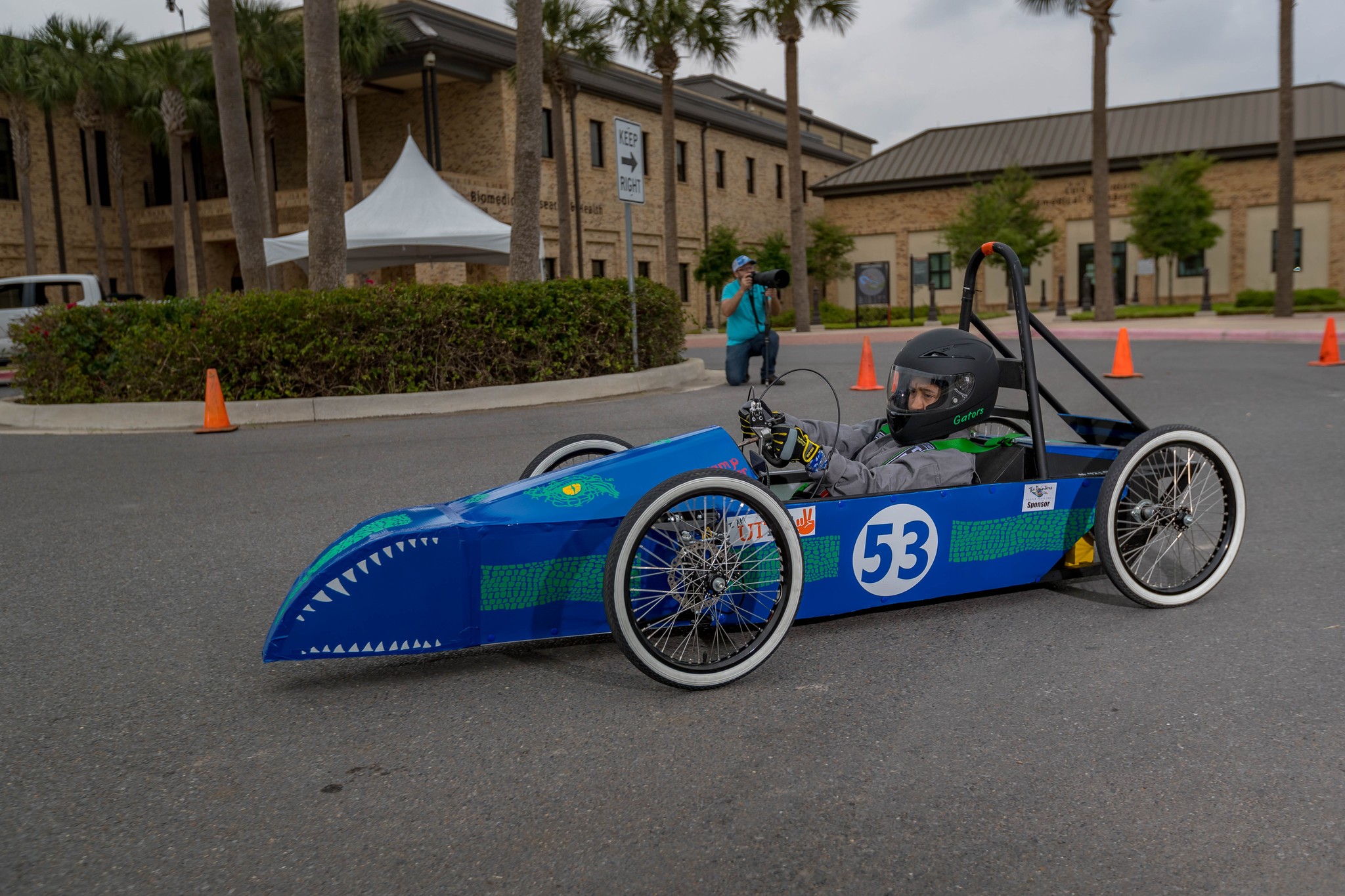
[[613, 115, 645, 204]]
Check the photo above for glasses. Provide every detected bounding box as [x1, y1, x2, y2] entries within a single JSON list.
[[736, 265, 756, 271]]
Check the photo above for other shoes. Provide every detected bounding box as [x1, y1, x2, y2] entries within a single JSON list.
[[741, 363, 750, 383], [760, 375, 785, 385]]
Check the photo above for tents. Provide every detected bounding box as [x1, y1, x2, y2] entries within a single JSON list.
[[264, 134, 545, 292]]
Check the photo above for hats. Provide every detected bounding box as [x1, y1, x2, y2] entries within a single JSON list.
[[731, 255, 757, 272]]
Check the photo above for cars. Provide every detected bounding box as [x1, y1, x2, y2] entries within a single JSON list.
[[261, 240, 1247, 690]]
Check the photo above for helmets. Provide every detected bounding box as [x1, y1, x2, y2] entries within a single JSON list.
[[885, 328, 999, 445]]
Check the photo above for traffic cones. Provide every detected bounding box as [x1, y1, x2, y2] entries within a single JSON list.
[[849, 335, 886, 391], [1102, 327, 1144, 378], [1308, 316, 1345, 367], [194, 368, 240, 434]]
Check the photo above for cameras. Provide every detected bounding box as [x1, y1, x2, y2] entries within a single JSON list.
[[749, 269, 791, 288]]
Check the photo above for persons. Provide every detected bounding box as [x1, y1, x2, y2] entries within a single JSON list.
[[721, 255, 786, 387], [738, 327, 999, 497]]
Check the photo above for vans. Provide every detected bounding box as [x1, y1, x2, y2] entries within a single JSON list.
[[0, 273, 148, 367]]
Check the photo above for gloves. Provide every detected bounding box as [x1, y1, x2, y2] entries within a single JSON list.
[[772, 423, 820, 465], [737, 398, 786, 439]]
